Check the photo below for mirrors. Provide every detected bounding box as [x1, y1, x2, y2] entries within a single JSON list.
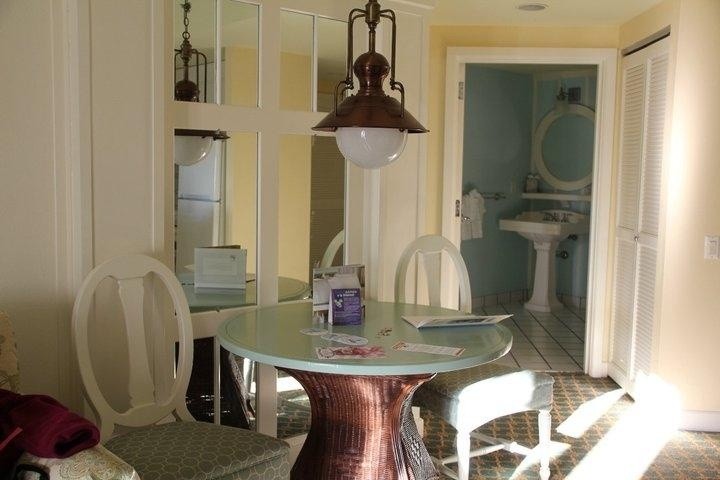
[[174, 0, 259, 107], [172, 129, 259, 315], [275, 369, 313, 440], [279, 9, 350, 117], [278, 133, 347, 302], [175, 336, 258, 432]]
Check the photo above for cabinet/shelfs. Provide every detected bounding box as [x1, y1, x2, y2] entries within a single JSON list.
[[608, 26, 671, 403]]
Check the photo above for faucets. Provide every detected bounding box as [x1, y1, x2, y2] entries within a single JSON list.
[[539, 211, 560, 222]]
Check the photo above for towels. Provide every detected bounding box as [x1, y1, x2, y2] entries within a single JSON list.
[[462, 189, 486, 241]]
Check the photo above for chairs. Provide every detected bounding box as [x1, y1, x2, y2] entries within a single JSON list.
[[392, 232, 555, 480], [0, 308, 141, 480], [321, 230, 345, 268], [71, 252, 293, 480]]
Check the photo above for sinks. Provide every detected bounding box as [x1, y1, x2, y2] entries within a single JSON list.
[[498, 209, 590, 242]]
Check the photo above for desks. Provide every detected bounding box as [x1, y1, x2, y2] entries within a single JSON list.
[[173, 272, 312, 431], [216, 298, 514, 480]]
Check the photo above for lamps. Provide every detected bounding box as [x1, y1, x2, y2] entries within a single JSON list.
[[311, 0, 430, 170], [174, 0, 230, 166]]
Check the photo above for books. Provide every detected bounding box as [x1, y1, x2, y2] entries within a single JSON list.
[[400, 312, 515, 330]]
